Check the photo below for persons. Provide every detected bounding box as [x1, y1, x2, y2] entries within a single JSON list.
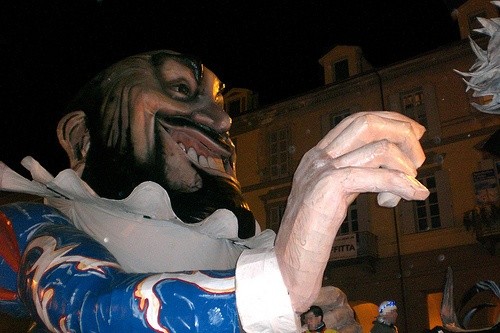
[[302, 305, 338, 333], [370, 300, 400, 333], [0, 48, 430, 333]]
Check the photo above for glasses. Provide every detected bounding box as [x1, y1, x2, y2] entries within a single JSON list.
[[380, 301, 396, 313]]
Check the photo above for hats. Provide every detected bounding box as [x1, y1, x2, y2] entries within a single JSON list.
[[378, 300, 397, 316]]
